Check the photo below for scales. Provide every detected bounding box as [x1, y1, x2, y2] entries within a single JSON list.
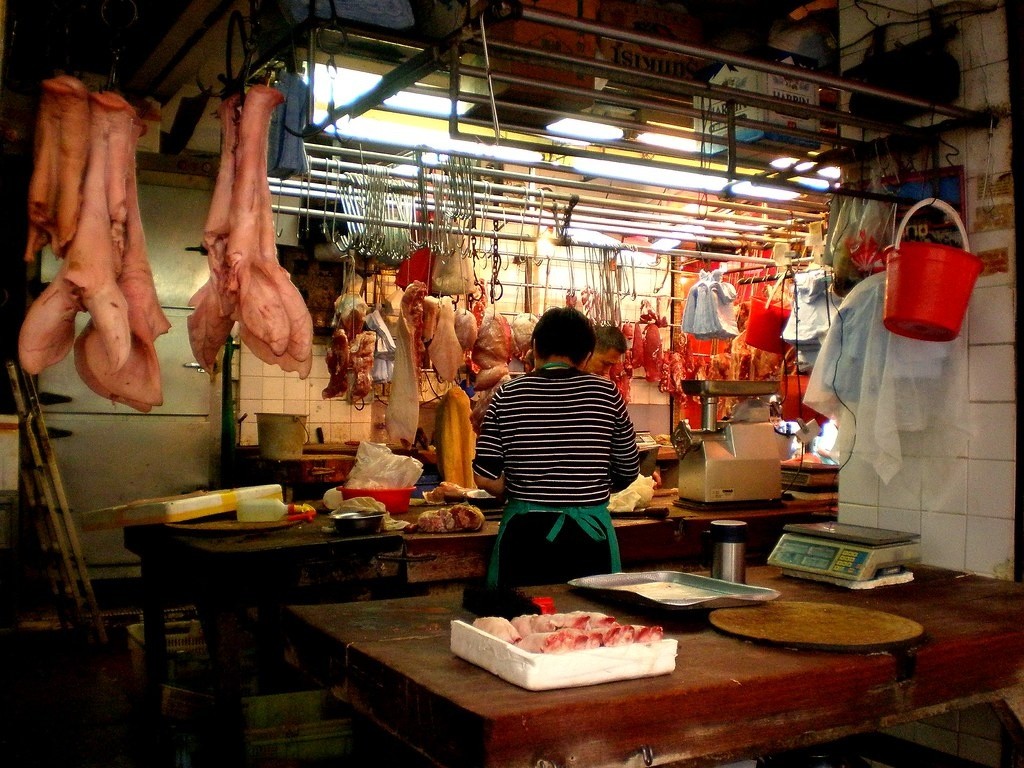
[[767, 519, 924, 582]]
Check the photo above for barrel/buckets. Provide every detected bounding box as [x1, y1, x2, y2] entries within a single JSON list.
[[254, 412, 309, 458], [882, 197, 985, 342], [745, 274, 792, 355]]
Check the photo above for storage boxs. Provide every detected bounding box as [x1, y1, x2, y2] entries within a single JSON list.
[[0, 414, 19, 491], [158, 683, 216, 723], [126, 619, 216, 688], [457, 0, 706, 130], [693, 65, 820, 166], [242, 658, 354, 764]]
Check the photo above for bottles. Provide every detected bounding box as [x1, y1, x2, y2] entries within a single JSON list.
[[237, 499, 295, 521]]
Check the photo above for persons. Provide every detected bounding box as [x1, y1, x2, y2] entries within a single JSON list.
[[769, 401, 781, 423], [471, 308, 640, 587], [585, 326, 627, 378]]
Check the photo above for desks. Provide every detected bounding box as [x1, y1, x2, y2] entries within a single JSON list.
[[236, 436, 680, 504], [282, 564, 1024, 768], [122, 488, 838, 689]]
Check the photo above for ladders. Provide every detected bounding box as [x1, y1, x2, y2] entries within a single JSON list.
[[6, 361, 109, 646]]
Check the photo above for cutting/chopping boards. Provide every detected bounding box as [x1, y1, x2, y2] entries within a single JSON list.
[[710, 602, 924, 652]]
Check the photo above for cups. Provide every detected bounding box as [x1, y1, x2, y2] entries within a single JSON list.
[[701, 520, 748, 583]]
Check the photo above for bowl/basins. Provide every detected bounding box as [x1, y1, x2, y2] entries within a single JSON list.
[[338, 486, 416, 513], [332, 512, 383, 534]]
